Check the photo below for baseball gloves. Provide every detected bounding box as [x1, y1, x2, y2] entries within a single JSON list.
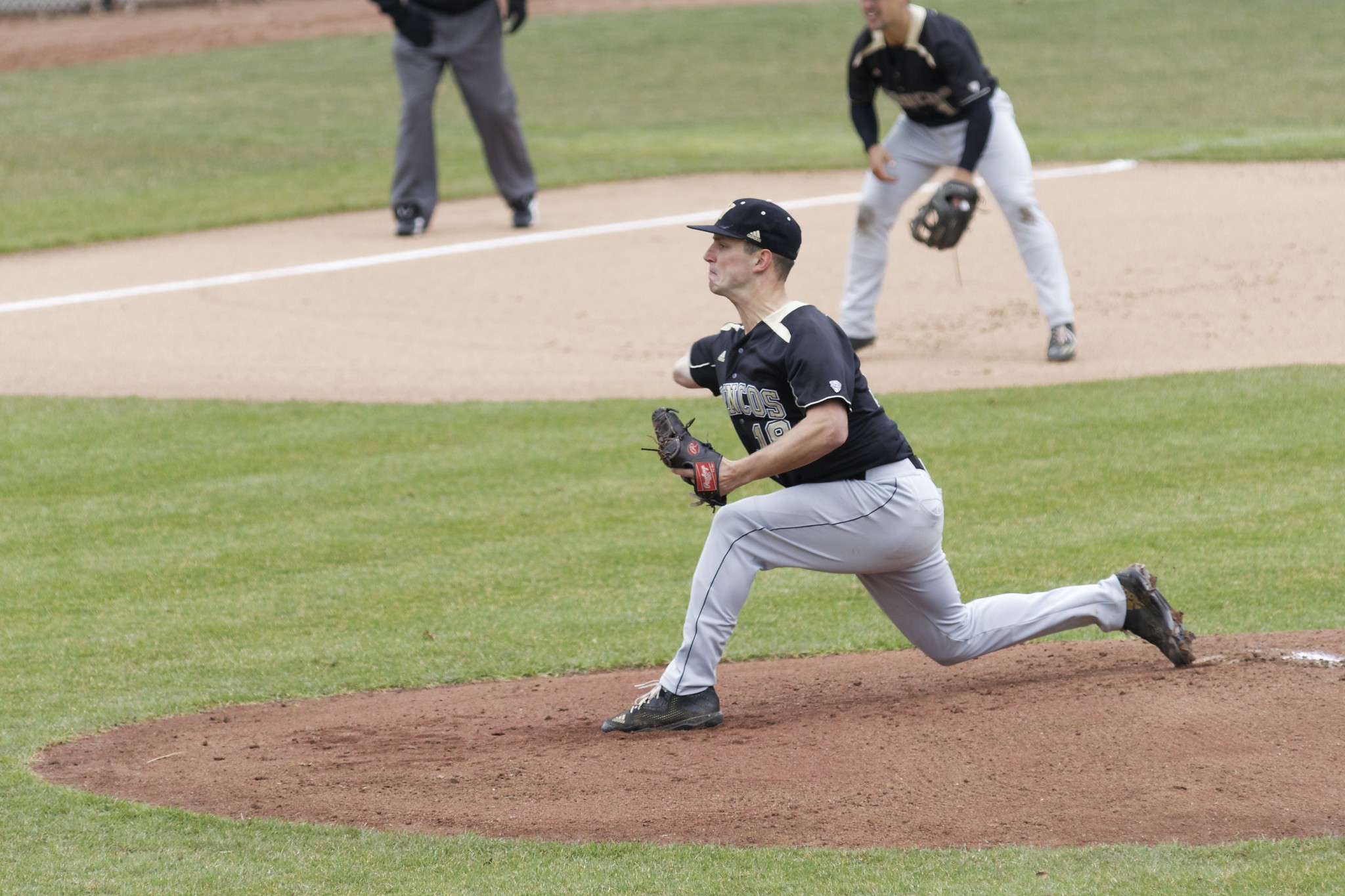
[[912, 180, 979, 249], [652, 406, 727, 507]]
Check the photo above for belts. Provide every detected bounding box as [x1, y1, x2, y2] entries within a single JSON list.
[[849, 455, 923, 480]]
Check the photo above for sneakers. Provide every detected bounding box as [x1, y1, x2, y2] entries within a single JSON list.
[[512, 208, 532, 228], [850, 336, 873, 353], [395, 205, 429, 237], [1118, 564, 1195, 667], [1047, 324, 1077, 361], [600, 680, 723, 732]]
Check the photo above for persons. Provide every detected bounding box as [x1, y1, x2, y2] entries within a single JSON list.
[[600, 198, 1196, 732], [838, 1, 1078, 362], [367, 0, 537, 236]]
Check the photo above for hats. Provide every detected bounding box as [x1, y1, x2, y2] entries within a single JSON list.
[[688, 198, 802, 261]]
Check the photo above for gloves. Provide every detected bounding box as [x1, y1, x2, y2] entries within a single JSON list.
[[505, 0, 526, 33], [392, 6, 434, 48]]
